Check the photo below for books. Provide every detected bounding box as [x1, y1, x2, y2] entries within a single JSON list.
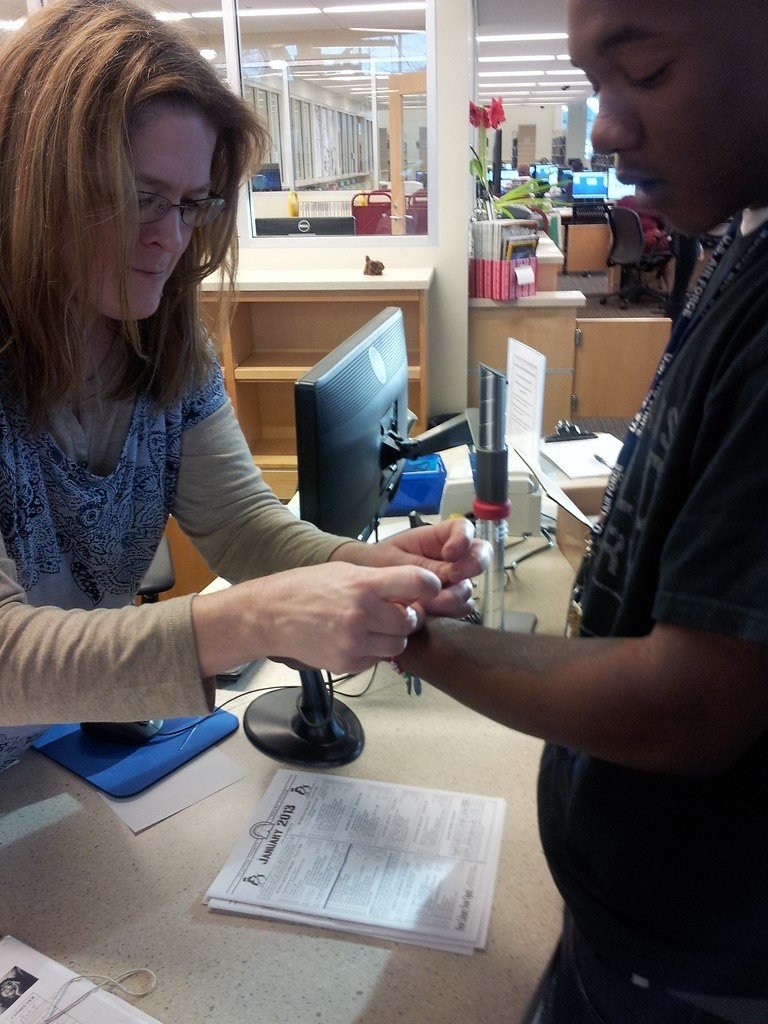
[[471, 219, 541, 260]]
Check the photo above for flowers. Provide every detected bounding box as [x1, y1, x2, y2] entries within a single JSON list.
[[468, 95, 575, 225]]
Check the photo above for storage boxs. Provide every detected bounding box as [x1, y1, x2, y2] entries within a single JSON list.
[[469, 256, 537, 300], [515, 448, 604, 574]]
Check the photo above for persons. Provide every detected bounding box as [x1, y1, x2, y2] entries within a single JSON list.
[[569, 160, 584, 172], [0, 0, 493, 725], [615, 183, 679, 297], [516, 165, 540, 193], [0, 978, 26, 1006], [378, 1, 768, 1024], [540, 158, 550, 165]]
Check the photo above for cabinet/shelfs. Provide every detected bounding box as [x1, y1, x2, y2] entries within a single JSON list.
[[150, 259, 434, 618], [467, 291, 673, 444]]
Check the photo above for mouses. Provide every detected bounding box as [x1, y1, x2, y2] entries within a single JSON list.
[[80, 718, 164, 745]]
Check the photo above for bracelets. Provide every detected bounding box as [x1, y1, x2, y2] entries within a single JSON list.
[[387, 656, 423, 697]]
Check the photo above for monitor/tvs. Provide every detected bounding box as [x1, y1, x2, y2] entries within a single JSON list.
[[534, 164, 561, 184], [571, 170, 610, 204], [293, 303, 472, 543], [250, 163, 282, 192]]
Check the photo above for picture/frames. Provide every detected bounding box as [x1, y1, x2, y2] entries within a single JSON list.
[[506, 240, 538, 261]]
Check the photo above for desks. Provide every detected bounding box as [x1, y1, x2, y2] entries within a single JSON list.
[[536, 230, 566, 291]]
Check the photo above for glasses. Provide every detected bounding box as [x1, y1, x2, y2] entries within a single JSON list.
[[99, 190, 227, 227]]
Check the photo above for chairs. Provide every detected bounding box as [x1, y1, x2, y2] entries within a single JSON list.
[[596, 207, 675, 310]]
